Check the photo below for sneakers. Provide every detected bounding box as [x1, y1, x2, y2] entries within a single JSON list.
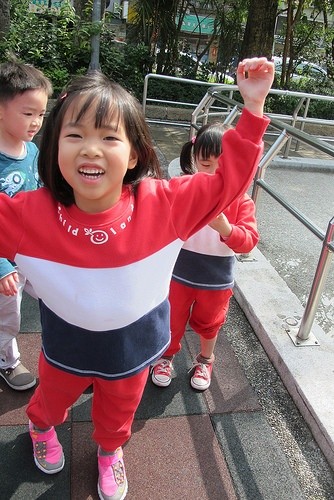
[[28, 418, 65, 474], [188, 362, 213, 391], [150, 357, 174, 387], [0, 358, 37, 390], [97, 444, 128, 500]]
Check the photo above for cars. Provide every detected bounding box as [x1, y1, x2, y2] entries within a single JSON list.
[[142, 46, 234, 87], [265, 58, 329, 88]]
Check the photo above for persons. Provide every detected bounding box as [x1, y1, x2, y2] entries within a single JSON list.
[[0, 57, 275, 500], [149, 123, 260, 391], [0, 63, 52, 392]]
